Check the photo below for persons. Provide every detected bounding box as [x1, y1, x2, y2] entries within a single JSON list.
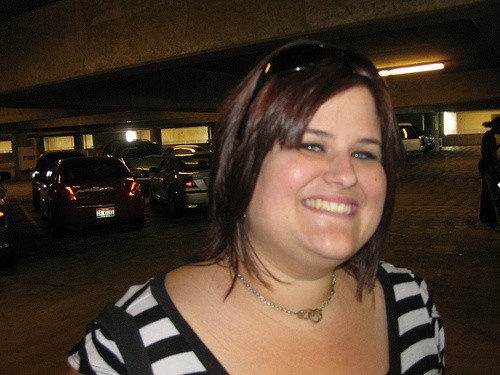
[[65, 38, 447, 375], [478, 119, 500, 227]]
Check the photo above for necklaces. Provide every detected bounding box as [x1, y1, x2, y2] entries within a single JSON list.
[[225, 256, 337, 324]]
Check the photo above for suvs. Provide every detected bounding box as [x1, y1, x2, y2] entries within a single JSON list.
[[397, 123, 435, 158], [101, 138, 213, 212]]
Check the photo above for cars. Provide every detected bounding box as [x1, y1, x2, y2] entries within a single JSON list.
[[26, 148, 84, 200], [0, 170, 11, 263], [33, 155, 145, 238]]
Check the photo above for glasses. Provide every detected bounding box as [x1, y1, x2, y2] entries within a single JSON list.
[[236, 52, 388, 143]]
